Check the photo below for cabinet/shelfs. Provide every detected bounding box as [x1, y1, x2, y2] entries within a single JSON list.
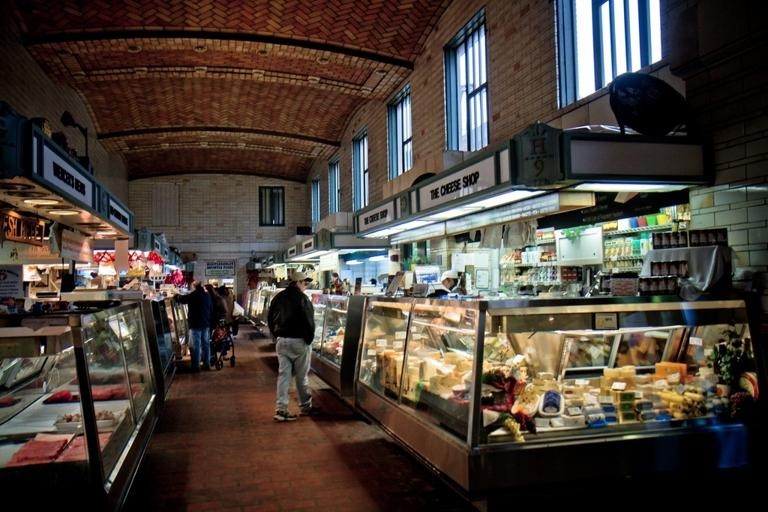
[[0, 298, 158, 508], [247, 289, 357, 402], [159, 295, 191, 398], [360, 296, 759, 490]]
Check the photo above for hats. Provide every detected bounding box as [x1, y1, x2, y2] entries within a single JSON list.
[[291, 272, 312, 282], [440, 270, 458, 282]]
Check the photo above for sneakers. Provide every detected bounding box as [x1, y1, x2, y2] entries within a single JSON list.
[[298, 401, 312, 416], [272, 411, 297, 421]]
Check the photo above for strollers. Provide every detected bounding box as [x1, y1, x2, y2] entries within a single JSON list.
[[210, 315, 241, 370]]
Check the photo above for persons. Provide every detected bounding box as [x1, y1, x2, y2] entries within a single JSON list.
[[170, 279, 234, 372], [330, 272, 344, 295], [426, 270, 460, 299], [266, 271, 316, 422]]
